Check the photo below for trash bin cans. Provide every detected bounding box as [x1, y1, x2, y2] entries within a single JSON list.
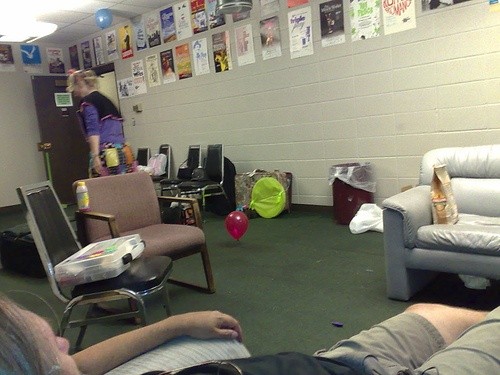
[[329, 163, 373, 224]]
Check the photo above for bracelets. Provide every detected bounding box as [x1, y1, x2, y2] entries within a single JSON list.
[[90, 152, 98, 157]]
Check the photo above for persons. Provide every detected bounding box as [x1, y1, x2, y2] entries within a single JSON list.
[[67, 70, 136, 178], [0, 294, 500, 375]]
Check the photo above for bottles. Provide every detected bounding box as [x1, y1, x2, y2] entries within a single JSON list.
[[76, 182, 90, 210]]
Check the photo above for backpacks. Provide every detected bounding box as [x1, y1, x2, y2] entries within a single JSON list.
[[148, 153, 167, 177], [0, 229, 47, 280]]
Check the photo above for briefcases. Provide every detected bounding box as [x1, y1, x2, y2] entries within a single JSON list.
[[53, 233, 146, 288], [234, 169, 290, 216]]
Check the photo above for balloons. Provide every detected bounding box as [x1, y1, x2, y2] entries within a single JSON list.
[[225, 211, 248, 241], [95, 9, 113, 30]]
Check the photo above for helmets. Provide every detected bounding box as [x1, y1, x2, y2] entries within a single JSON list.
[[192, 167, 209, 181]]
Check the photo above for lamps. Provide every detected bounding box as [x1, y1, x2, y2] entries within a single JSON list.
[[215, 0, 253, 14]]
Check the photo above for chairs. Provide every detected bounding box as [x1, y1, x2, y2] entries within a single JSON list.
[[382, 145, 499, 302], [72, 169, 216, 327], [16, 181, 173, 353], [136, 143, 233, 225]]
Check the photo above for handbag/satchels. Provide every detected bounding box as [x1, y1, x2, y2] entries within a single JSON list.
[[176, 156, 193, 179], [162, 206, 183, 225], [430, 163, 459, 225]]
